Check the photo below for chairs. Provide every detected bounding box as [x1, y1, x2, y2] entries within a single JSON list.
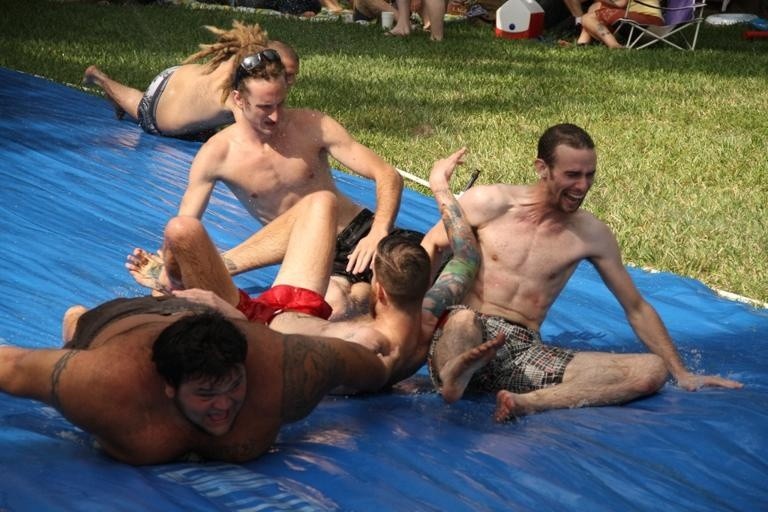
[[607, 0, 708, 53]]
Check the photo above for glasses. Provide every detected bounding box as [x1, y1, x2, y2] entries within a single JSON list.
[[235, 49, 281, 79]]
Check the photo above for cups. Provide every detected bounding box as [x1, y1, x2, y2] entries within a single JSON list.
[[381, 11, 395, 29]]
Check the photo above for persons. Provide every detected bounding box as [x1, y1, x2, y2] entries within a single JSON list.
[[300, 0, 665, 55], [154, 24, 448, 333], [416, 123, 747, 426], [119, 146, 484, 400], [78, 37, 299, 141], [0, 290, 398, 471]]
